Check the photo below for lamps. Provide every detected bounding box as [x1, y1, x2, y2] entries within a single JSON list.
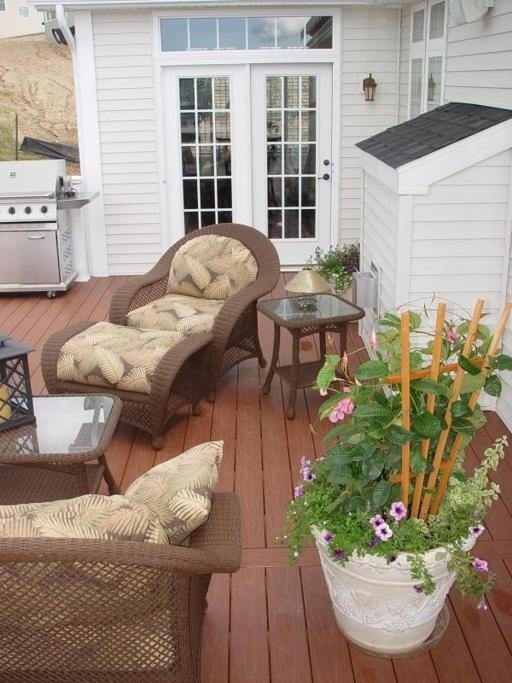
[[284, 267, 331, 311], [362, 78, 377, 102]]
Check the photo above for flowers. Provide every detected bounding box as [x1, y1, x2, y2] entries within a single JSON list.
[[272, 295, 512, 612]]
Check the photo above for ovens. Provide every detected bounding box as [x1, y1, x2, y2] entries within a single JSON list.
[[0, 159, 100, 298]]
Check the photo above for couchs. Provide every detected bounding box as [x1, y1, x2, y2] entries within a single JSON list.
[[41, 320, 214, 450], [0, 492, 243, 683], [108, 223, 280, 404]]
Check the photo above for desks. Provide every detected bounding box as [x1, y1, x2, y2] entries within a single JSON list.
[[0, 393, 123, 503]]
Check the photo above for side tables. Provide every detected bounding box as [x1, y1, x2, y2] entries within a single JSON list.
[[257, 293, 365, 420]]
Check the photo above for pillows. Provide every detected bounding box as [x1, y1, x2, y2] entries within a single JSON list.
[[124, 440, 225, 545]]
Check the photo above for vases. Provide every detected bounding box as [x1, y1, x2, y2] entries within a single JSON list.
[[308, 522, 477, 653]]
